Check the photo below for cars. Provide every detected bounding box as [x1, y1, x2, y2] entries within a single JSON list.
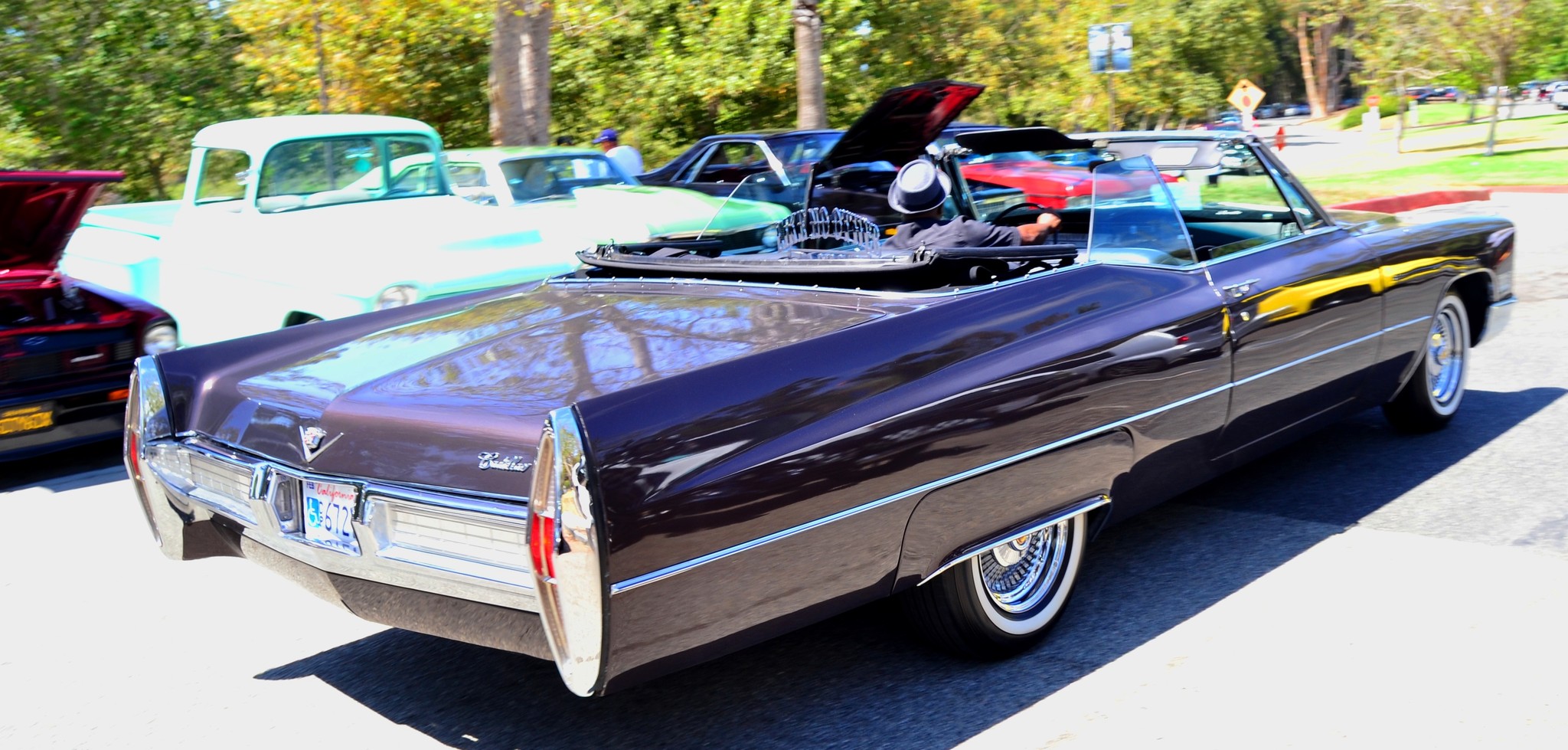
[[344, 144, 798, 279], [1201, 80, 1568, 132], [54, 110, 585, 360], [0, 168, 184, 475], [555, 125, 894, 212]]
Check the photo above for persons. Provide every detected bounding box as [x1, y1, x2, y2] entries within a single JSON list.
[[592, 129, 646, 179], [881, 160, 1060, 248]]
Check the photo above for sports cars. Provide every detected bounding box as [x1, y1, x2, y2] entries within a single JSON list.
[[119, 120, 1520, 704]]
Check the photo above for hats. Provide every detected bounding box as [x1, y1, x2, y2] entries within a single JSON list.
[[887, 158, 952, 213], [593, 128, 617, 144]]
